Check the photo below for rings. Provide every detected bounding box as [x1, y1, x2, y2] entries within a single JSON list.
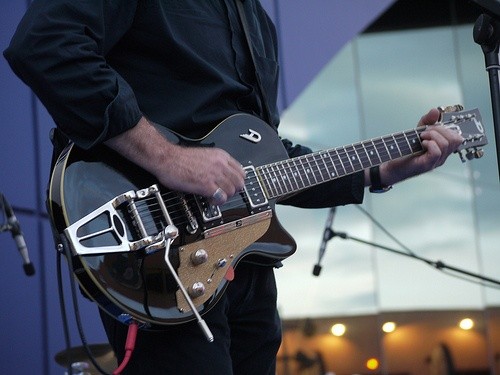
[[212, 187, 223, 202]]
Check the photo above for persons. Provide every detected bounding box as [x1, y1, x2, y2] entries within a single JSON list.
[[2, 1, 463, 375]]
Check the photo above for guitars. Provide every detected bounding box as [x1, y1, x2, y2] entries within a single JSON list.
[[46, 105, 489, 331]]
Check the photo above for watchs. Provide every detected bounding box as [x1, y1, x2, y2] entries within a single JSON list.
[[369, 166, 393, 194]]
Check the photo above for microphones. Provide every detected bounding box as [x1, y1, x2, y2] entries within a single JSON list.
[[312, 207, 336, 276], [2, 194, 36, 276]]
